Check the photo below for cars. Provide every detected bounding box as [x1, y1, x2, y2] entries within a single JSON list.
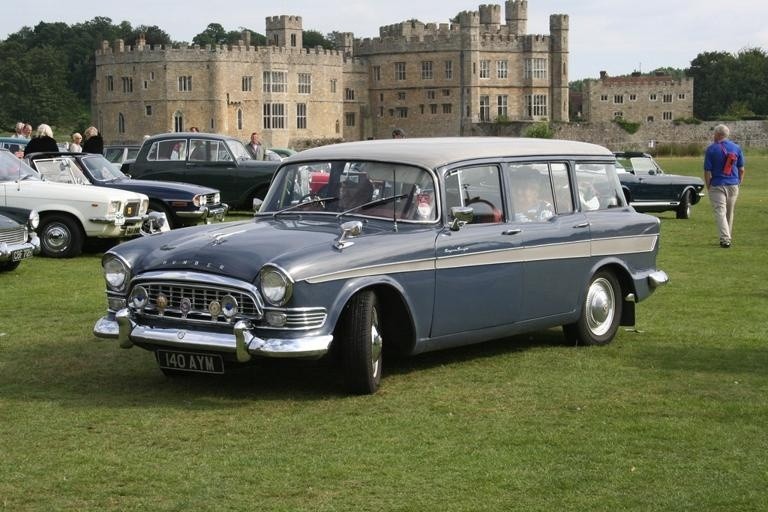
[[89, 135, 668, 388]]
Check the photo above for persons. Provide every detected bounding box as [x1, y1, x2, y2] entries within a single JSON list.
[[23, 122, 62, 160], [705, 124, 745, 247], [567, 172, 601, 212], [11, 122, 28, 139], [81, 127, 106, 156], [245, 132, 264, 160], [513, 166, 553, 224], [188, 141, 212, 160], [68, 132, 84, 153], [22, 124, 33, 139], [190, 126, 200, 133]]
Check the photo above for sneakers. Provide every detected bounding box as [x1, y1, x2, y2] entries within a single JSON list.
[[720, 241, 731, 248]]
